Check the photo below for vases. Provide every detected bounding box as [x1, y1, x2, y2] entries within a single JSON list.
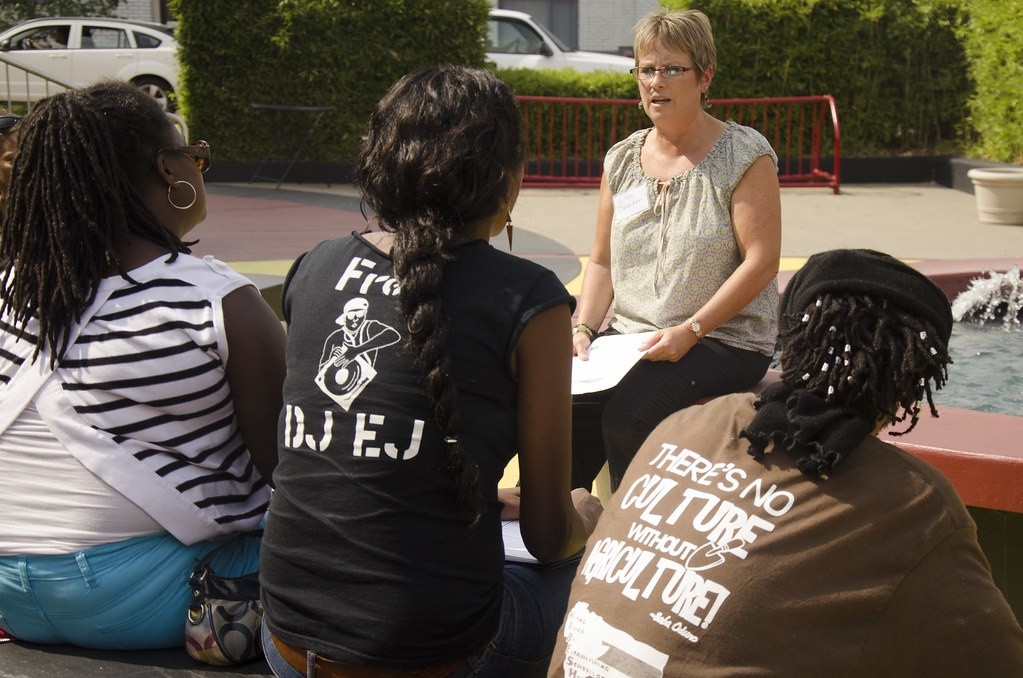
[[967, 168, 1023, 224]]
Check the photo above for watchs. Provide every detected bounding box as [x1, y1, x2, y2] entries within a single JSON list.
[[686, 317, 704, 341]]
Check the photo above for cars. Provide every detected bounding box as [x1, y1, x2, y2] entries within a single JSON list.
[[0, 17, 187, 115], [480, 7, 636, 78]]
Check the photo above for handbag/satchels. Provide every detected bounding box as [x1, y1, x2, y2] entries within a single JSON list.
[[184, 531, 265, 667]]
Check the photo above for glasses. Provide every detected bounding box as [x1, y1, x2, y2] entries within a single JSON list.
[[152, 139, 211, 173], [630, 65, 698, 79]]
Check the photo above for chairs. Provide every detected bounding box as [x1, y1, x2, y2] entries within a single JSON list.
[[82, 31, 95, 48]]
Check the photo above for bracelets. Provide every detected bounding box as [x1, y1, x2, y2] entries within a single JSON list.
[[572, 321, 597, 342]]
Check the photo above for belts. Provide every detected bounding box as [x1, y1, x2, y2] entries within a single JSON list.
[[270, 633, 465, 678]]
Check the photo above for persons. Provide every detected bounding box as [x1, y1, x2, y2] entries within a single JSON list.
[[569, 11, 782, 496], [258, 67, 606, 677], [548, 249, 1023, 678], [0, 80, 289, 651], [31, 27, 67, 49]]
